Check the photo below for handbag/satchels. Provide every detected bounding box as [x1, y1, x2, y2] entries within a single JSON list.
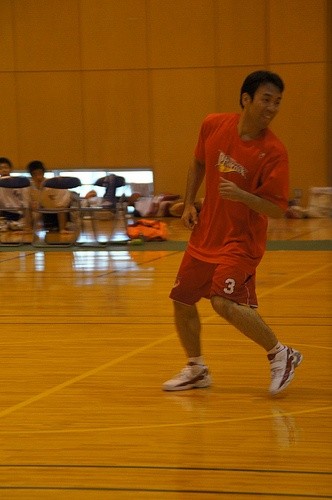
[[127, 219, 169, 241]]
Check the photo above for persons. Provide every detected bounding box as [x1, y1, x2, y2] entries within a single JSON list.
[[0, 157, 72, 243], [162, 70, 302, 395]]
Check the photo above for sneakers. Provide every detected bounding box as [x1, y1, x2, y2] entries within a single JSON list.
[[267, 345, 304, 396], [162, 365, 211, 391]]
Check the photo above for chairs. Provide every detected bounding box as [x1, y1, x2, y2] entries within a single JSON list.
[[0, 176, 32, 246], [35, 176, 81, 245]]
